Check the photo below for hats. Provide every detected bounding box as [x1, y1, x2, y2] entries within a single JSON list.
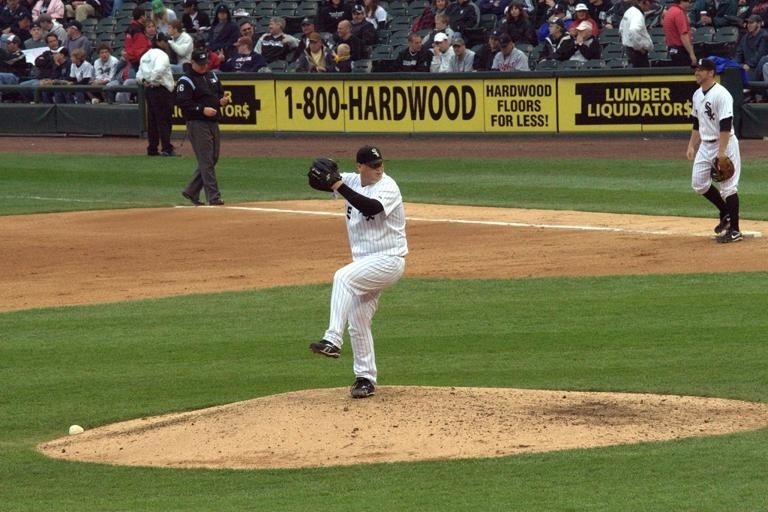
[[301, 17, 314, 25], [357, 145, 384, 165], [575, 21, 593, 31], [233, 37, 252, 46], [545, 17, 564, 27], [434, 33, 447, 42], [3, 35, 21, 44], [351, 5, 364, 13], [152, 31, 172, 40], [308, 32, 321, 42], [151, 1, 163, 15], [549, 2, 589, 11], [50, 47, 67, 56], [745, 15, 763, 22], [191, 50, 210, 65], [489, 29, 511, 47], [453, 38, 465, 47], [64, 19, 83, 30], [691, 59, 716, 70]]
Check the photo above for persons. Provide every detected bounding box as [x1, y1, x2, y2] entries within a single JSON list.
[[393, 1, 611, 72], [285, 0, 389, 74], [174, 52, 229, 205], [0, 1, 140, 104], [134, 31, 182, 157], [686, 58, 743, 243], [309, 144, 410, 398], [603, 0, 768, 81], [123, 1, 303, 74]]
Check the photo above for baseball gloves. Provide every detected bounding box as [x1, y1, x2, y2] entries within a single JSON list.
[[711, 157, 734, 182], [308, 157, 342, 192]]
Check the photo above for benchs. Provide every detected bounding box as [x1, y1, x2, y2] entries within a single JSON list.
[[0, 0, 768, 71]]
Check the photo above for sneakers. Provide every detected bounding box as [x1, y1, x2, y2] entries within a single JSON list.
[[209, 199, 224, 205], [309, 340, 341, 357], [163, 150, 182, 156], [351, 378, 375, 399], [717, 230, 742, 243], [182, 191, 205, 205], [714, 215, 731, 233]]
